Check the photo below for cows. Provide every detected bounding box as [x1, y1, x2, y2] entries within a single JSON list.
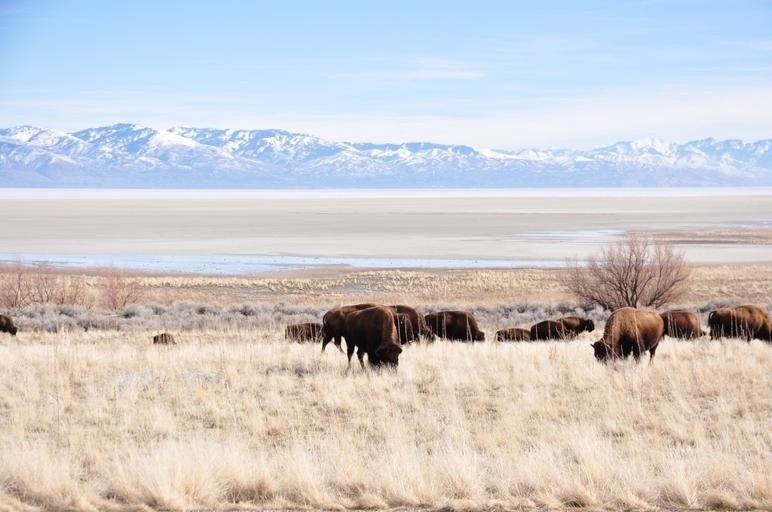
[[284, 303, 486, 355], [707, 304, 772, 343], [658, 309, 707, 341], [345, 305, 403, 370], [590, 306, 665, 366], [153, 333, 177, 345], [0, 315, 18, 336], [494, 316, 595, 342]]
